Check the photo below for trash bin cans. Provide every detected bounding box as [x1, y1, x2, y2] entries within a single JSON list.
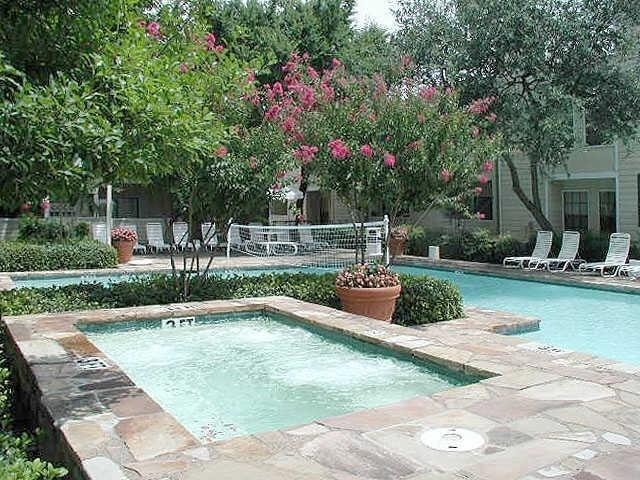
[[366, 227, 381, 255]]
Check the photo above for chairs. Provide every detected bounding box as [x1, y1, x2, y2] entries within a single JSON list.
[[502, 230, 639, 283], [91, 219, 315, 256]]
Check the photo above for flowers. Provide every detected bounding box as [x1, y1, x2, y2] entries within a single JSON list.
[[389, 227, 408, 242], [336, 258, 399, 289]]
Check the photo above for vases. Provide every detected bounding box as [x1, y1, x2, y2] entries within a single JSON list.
[[336, 285, 401, 325], [391, 240, 404, 255], [112, 241, 135, 264]]
